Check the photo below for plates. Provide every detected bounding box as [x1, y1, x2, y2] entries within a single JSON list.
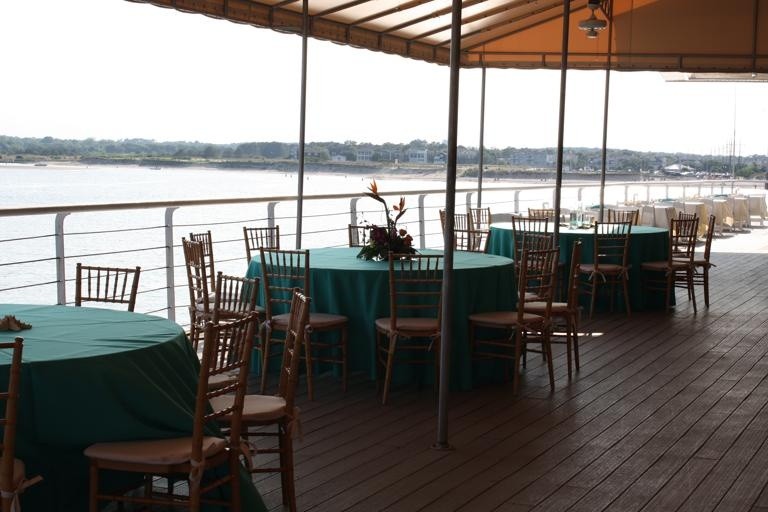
[[559, 223, 570, 226]]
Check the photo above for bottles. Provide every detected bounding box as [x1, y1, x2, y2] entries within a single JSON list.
[[569, 202, 595, 229]]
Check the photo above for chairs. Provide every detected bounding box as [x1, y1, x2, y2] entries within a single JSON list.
[[179, 223, 584, 404], [438, 191, 766, 321], [0, 259, 314, 510]]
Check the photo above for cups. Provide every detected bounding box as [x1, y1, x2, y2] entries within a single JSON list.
[[564, 214, 570, 223], [559, 215, 564, 223]]
